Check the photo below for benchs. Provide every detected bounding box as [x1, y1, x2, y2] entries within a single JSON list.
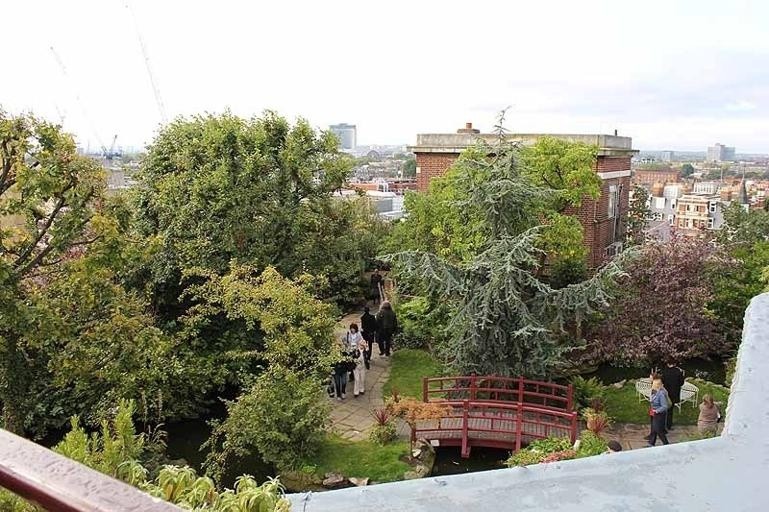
[[635, 378, 699, 414]]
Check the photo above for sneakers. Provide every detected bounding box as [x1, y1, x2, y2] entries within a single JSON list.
[[337, 392, 347, 401], [379, 353, 391, 356]]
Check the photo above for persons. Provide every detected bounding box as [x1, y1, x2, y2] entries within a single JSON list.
[[643, 360, 684, 448], [333, 269, 397, 401], [601, 440, 622, 455], [697, 393, 721, 438]]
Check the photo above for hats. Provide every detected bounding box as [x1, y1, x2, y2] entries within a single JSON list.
[[363, 306, 370, 312]]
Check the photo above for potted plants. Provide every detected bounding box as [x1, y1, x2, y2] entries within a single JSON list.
[[581, 395, 611, 430]]
